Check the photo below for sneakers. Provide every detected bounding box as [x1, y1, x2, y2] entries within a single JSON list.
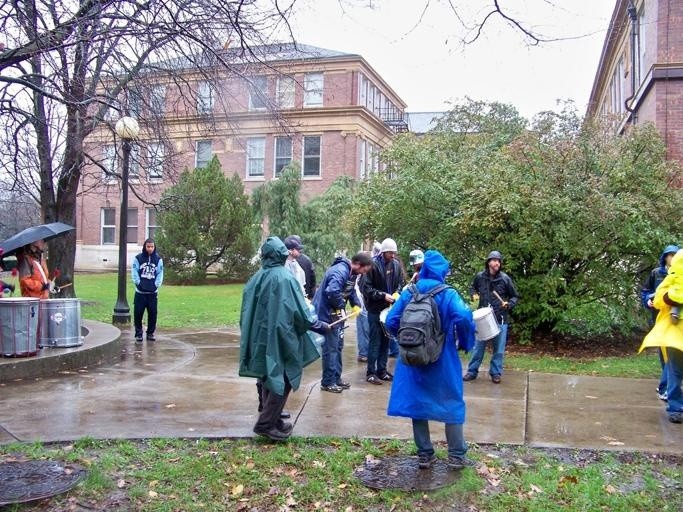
[[320, 384, 343, 393], [254, 428, 289, 440], [463, 374, 476, 381], [419, 454, 438, 468], [448, 457, 477, 468], [136, 335, 143, 342], [367, 376, 385, 385], [280, 422, 292, 434], [672, 313, 679, 325], [492, 376, 501, 384], [337, 380, 351, 389], [147, 333, 155, 341], [281, 412, 290, 418], [669, 412, 683, 423], [358, 355, 368, 362], [382, 371, 393, 381]]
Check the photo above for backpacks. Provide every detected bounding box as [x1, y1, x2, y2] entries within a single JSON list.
[[397, 283, 456, 367]]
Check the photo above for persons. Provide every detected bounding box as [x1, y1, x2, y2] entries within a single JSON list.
[[132, 238, 163, 344], [401, 250, 425, 291], [641, 244, 679, 401], [354, 240, 381, 363], [463, 251, 518, 384], [295, 235, 317, 300], [311, 252, 373, 394], [239, 237, 332, 439], [284, 235, 325, 359], [19, 239, 60, 350], [358, 237, 405, 385], [384, 250, 476, 468], [637, 248, 683, 423]]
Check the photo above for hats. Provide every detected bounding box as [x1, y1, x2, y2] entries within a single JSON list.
[[409, 250, 424, 265], [285, 235, 301, 244], [380, 238, 397, 253], [285, 239, 304, 250]]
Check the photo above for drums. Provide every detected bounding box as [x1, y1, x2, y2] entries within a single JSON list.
[[472, 307, 501, 341], [378, 306, 396, 339], [38, 297, 82, 346], [0, 297, 39, 357]]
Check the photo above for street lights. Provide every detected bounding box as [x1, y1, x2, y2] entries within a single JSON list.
[[112, 116, 139, 323]]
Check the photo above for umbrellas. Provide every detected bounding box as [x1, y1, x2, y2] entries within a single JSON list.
[[1, 222, 77, 260]]
